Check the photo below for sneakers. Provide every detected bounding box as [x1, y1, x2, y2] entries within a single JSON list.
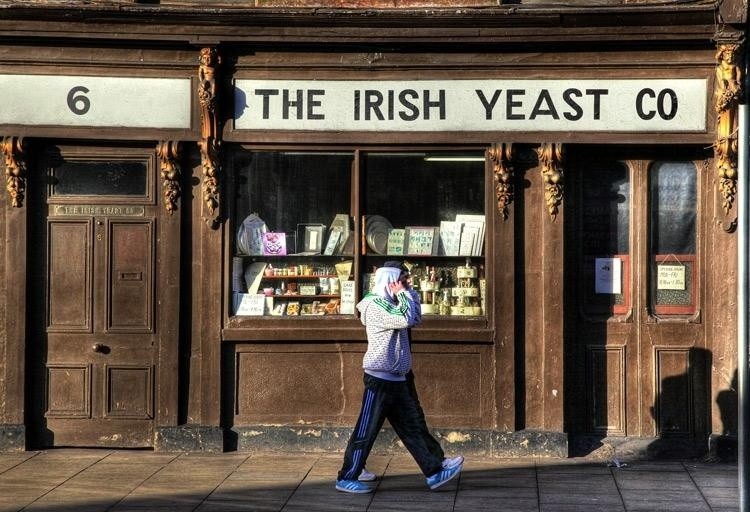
[[358, 468, 376, 481], [442, 455, 464, 469], [335, 475, 373, 494], [425, 464, 462, 490]]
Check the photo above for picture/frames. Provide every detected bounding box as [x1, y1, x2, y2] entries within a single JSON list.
[[585, 255, 629, 316], [651, 254, 697, 314]]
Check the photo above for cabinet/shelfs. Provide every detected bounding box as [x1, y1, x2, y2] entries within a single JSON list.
[[233, 250, 484, 319]]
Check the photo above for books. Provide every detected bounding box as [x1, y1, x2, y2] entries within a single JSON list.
[[437, 214, 486, 257]]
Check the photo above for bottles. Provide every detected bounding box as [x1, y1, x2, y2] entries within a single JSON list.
[[264, 263, 311, 277]]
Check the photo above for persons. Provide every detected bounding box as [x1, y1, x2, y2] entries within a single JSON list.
[[197, 46, 222, 139], [714, 44, 743, 170]]
